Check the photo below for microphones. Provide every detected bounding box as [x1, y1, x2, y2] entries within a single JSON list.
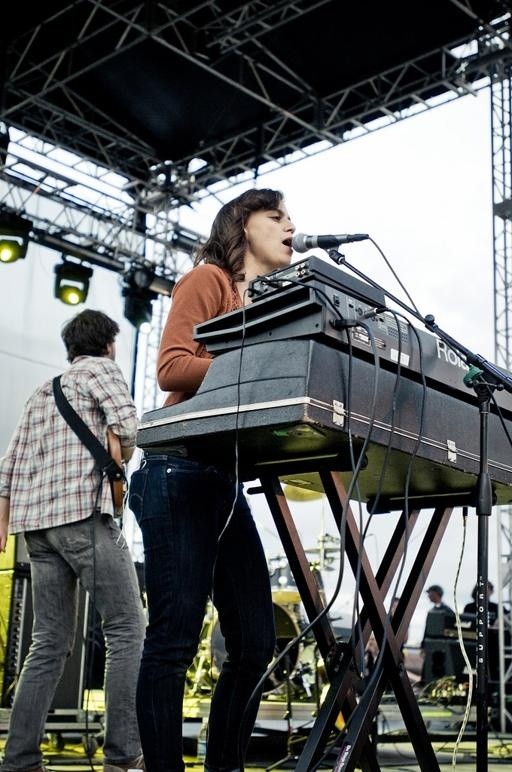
[[291, 233, 368, 253]]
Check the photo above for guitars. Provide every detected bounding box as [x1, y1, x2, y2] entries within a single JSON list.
[[108, 425, 125, 519]]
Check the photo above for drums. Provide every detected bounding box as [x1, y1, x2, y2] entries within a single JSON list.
[[193, 602, 304, 695]]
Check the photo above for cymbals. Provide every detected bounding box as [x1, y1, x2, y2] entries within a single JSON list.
[[270, 590, 304, 605]]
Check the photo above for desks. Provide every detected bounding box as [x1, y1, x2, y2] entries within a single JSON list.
[[136, 403, 512, 772]]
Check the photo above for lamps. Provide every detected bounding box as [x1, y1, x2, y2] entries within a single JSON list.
[[1, 229, 29, 262], [121, 289, 159, 329], [123, 265, 156, 290], [54, 261, 93, 306]]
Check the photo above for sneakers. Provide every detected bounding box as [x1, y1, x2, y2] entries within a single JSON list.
[[26, 766, 51, 771], [104, 754, 146, 772]]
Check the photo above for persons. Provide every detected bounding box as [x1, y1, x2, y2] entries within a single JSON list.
[[390, 594, 409, 656], [462, 577, 511, 628], [0, 308, 147, 772], [418, 585, 455, 690], [129, 187, 296, 770]]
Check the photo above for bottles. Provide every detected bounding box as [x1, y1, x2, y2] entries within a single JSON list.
[[197, 715, 208, 764]]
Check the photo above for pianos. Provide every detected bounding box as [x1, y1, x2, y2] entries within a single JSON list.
[[192, 279, 512, 420], [135, 339, 512, 507]]
